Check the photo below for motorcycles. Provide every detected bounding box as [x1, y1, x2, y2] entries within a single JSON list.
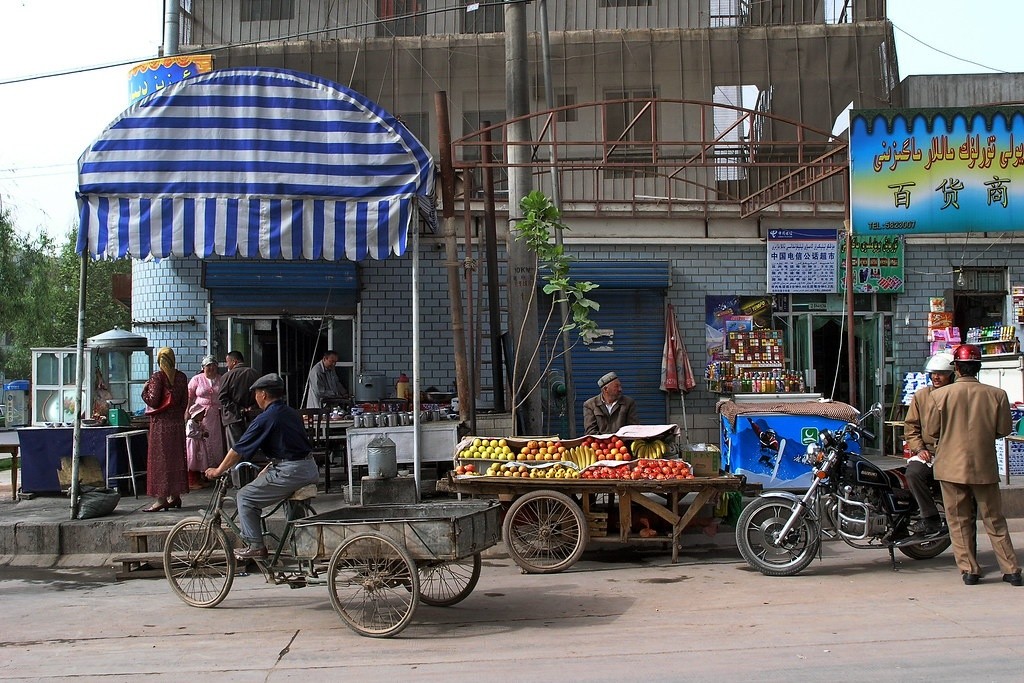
[[735, 401, 951, 578]]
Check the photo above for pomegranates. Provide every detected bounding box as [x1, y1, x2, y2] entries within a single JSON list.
[[582, 460, 695, 480]]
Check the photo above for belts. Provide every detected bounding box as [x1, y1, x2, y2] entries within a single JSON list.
[[272, 453, 312, 464]]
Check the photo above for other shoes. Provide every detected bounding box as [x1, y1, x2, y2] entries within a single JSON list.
[[287, 573, 308, 583], [1003, 573, 1022, 585], [235, 546, 268, 561], [962, 573, 979, 585], [189, 482, 209, 490], [907, 515, 942, 532]]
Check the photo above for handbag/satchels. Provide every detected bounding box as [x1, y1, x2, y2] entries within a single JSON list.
[[144, 372, 172, 416]]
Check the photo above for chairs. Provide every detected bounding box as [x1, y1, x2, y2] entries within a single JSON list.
[[242, 405, 331, 494]]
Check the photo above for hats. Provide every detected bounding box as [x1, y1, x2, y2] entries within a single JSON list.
[[202, 355, 218, 367], [597, 371, 618, 388], [188, 402, 206, 419], [249, 373, 284, 392]]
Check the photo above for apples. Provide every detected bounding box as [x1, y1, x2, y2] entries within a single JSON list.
[[580, 436, 631, 461], [455, 439, 580, 478]]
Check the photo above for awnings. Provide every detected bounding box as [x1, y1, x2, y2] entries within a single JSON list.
[[75, 67, 439, 259]]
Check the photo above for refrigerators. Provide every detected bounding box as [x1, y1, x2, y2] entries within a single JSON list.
[[719, 411, 860, 491]]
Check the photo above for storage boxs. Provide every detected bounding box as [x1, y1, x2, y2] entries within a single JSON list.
[[903, 299, 961, 406], [995, 410, 1024, 476], [902, 440, 916, 458], [680, 443, 719, 477]]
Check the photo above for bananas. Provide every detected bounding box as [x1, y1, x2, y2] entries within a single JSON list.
[[631, 440, 666, 459], [561, 446, 597, 469]]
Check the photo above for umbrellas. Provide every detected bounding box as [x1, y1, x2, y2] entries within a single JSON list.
[[658, 303, 695, 444]]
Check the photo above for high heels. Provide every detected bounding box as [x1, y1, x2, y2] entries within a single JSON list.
[[142, 500, 169, 512], [169, 497, 182, 508]]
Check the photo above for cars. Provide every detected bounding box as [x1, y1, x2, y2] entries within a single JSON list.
[[0, 405, 25, 427]]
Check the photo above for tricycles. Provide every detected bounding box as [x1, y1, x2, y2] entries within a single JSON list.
[[163, 463, 505, 640]]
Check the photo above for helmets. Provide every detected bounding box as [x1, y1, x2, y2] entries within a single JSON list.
[[925, 353, 954, 373], [950, 344, 981, 365], [766, 428, 775, 434]]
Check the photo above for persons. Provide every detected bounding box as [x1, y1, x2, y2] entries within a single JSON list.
[[140, 347, 188, 511], [748, 418, 780, 473], [578, 372, 637, 505], [203, 373, 321, 585], [904, 353, 977, 559], [184, 350, 262, 490], [924, 343, 1023, 586], [306, 351, 349, 409]]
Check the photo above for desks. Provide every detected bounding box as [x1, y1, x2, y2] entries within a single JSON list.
[[346, 420, 464, 501], [17, 425, 131, 494], [0, 443, 21, 500], [885, 421, 905, 456], [304, 420, 354, 439], [1005, 437, 1024, 484]]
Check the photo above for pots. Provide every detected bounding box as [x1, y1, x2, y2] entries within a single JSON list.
[[397, 470, 410, 476], [423, 392, 458, 403]]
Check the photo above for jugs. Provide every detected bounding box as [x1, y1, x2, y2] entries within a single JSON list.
[[330, 408, 344, 420]]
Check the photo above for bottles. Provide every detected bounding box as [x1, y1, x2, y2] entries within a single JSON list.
[[981, 343, 1014, 354], [967, 322, 1015, 343], [705, 360, 805, 392], [397, 374, 409, 411]]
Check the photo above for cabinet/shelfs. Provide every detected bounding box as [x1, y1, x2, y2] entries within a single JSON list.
[[966, 341, 1018, 361], [1013, 286, 1024, 324]]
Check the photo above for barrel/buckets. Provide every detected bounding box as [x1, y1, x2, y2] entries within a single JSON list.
[[368, 437, 397, 479], [355, 372, 386, 403], [903, 440, 915, 459]]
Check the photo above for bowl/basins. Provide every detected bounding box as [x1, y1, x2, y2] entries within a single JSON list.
[[54, 423, 62, 427], [82, 419, 96, 424], [66, 423, 71, 425], [45, 422, 54, 427], [440, 408, 457, 419]]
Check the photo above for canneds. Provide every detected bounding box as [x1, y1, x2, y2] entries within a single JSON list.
[[715, 361, 772, 382], [1000, 326, 1015, 339]]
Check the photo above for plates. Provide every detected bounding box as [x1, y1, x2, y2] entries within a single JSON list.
[[81, 423, 97, 426]]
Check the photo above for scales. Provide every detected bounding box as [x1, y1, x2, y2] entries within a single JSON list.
[[105, 398, 132, 427]]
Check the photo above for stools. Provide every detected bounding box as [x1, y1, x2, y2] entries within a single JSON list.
[[106, 429, 149, 499]]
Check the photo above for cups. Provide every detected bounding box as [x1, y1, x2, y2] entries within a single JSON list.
[[354, 412, 397, 427], [430, 411, 439, 422], [398, 410, 429, 426]]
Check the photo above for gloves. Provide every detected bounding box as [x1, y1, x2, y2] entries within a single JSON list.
[[747, 417, 753, 423]]
